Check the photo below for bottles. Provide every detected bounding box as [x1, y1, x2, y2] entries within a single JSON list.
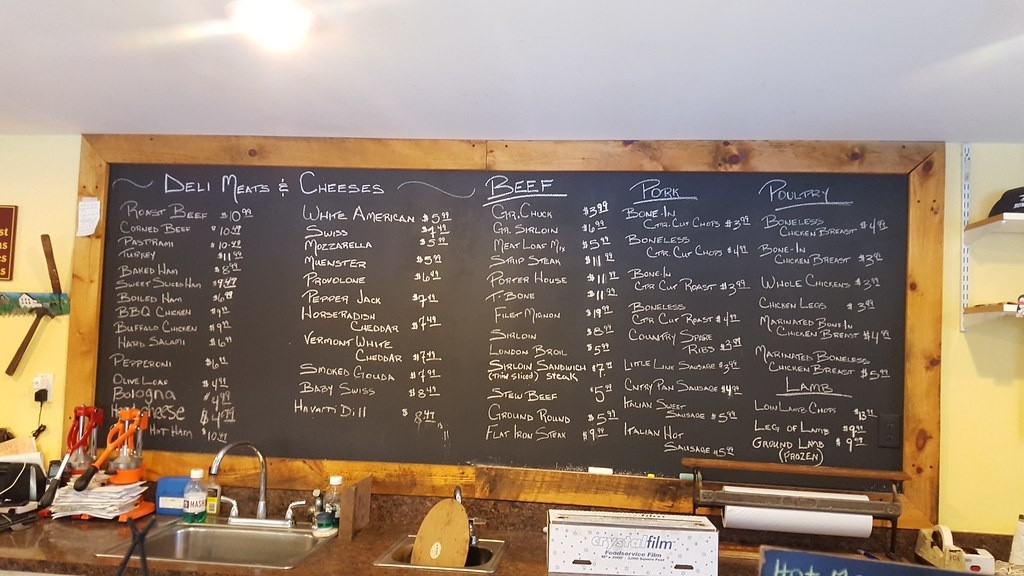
[[206, 466, 221, 516], [183, 468, 208, 523], [323, 475, 343, 525]]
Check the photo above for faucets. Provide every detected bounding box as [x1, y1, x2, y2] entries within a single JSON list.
[[450, 486, 478, 547], [210, 440, 269, 521]]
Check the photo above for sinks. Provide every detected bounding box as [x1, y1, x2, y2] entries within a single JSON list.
[[371, 532, 507, 574], [94, 515, 338, 570]]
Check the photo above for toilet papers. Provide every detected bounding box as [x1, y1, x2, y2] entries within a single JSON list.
[[720, 485, 874, 539]]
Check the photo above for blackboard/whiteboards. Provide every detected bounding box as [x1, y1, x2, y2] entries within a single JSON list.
[[57, 134, 939, 532]]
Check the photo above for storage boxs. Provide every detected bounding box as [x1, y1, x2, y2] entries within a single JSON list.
[[544, 507, 719, 575]]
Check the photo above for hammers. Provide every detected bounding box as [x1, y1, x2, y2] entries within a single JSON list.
[[4, 304, 55, 381]]
